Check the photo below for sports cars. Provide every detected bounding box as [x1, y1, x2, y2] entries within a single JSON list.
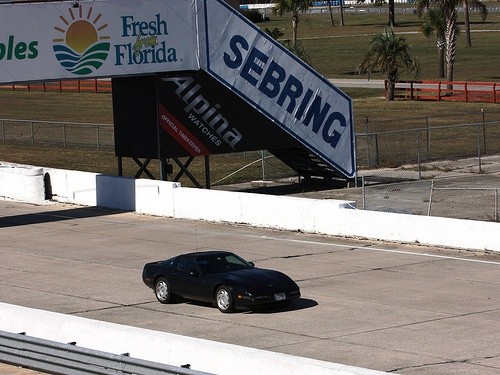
[[141, 248, 304, 314]]
